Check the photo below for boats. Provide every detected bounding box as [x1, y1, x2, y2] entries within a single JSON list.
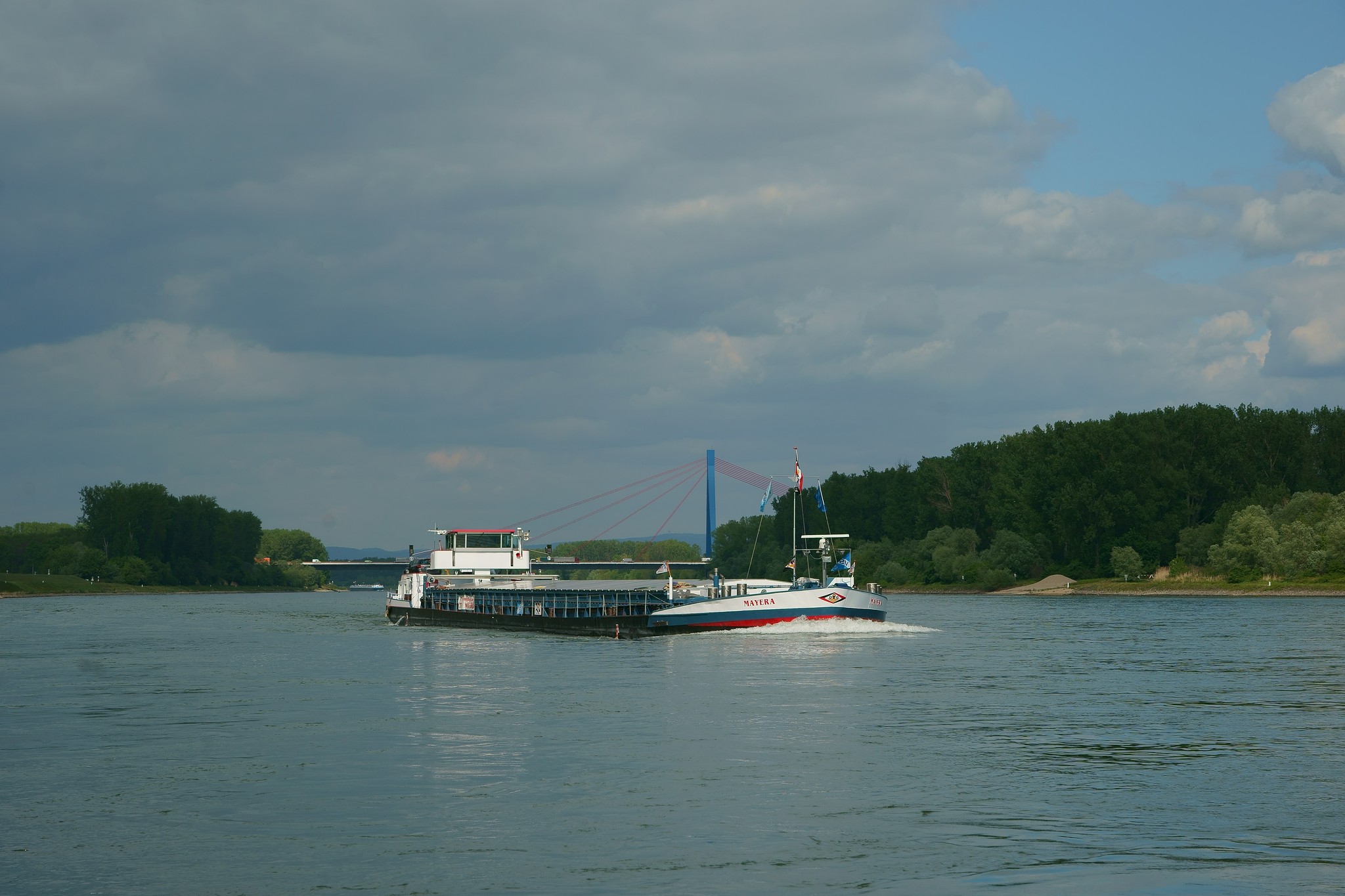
[[350, 581, 384, 591], [386, 447, 888, 640]]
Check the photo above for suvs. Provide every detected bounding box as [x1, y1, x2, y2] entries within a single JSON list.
[[662, 560, 669, 562]]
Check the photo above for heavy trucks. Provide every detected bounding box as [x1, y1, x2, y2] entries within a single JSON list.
[[254, 558, 270, 564]]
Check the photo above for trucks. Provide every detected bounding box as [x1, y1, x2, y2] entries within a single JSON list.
[[701, 558, 713, 562], [622, 558, 632, 563]]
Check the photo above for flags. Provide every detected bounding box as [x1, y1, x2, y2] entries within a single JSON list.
[[831, 552, 851, 571], [760, 480, 773, 512], [813, 485, 827, 513], [847, 561, 855, 573], [796, 450, 803, 492], [782, 553, 796, 572], [656, 561, 669, 574], [439, 542, 450, 575]]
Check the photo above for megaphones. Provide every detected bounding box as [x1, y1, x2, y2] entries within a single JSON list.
[[524, 532, 529, 536], [524, 537, 529, 541]]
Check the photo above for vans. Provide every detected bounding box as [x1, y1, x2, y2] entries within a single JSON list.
[[365, 560, 373, 563], [312, 559, 321, 563]]
